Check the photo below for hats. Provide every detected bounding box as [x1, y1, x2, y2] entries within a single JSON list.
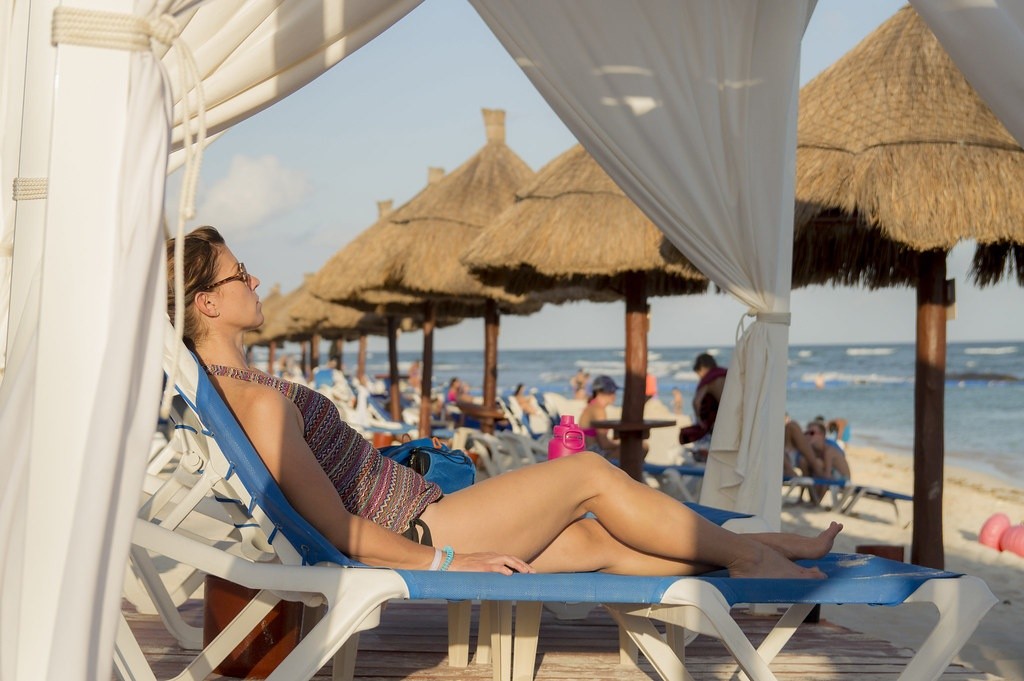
[[593, 376, 622, 392]]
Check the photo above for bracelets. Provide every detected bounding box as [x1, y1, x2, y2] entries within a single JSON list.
[[430, 546, 454, 571]]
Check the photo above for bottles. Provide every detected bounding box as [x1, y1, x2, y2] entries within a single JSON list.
[[547, 415, 587, 460]]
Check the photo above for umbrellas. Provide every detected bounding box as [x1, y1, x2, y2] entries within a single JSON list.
[[244, 105, 557, 437], [460, 132, 712, 480], [795, 0, 1023, 568]]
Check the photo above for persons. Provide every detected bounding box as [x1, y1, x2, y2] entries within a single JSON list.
[[571, 368, 589, 399], [689, 351, 851, 505], [167, 225, 845, 580], [513, 384, 534, 414], [446, 375, 473, 404], [814, 366, 829, 392], [311, 357, 339, 374], [579, 374, 649, 465], [671, 386, 683, 412]]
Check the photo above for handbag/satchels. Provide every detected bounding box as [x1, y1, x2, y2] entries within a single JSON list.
[[377, 433, 476, 494]]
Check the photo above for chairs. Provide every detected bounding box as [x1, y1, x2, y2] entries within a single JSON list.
[[108, 311, 998, 681]]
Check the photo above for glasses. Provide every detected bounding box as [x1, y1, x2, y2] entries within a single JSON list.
[[201, 263, 247, 291]]
